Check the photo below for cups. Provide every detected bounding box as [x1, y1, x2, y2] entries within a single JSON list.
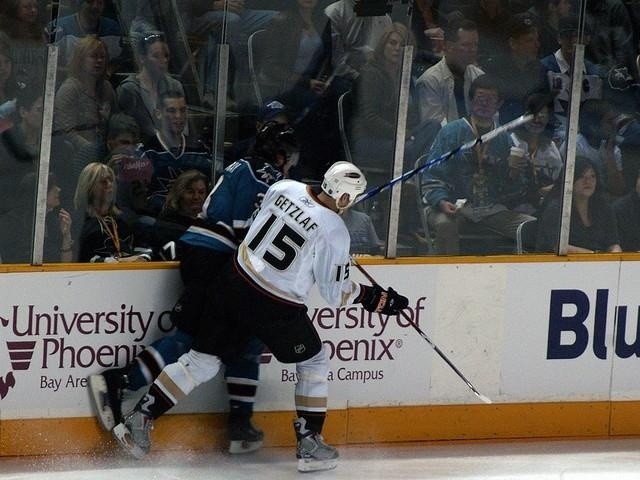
[[508, 146, 525, 169]]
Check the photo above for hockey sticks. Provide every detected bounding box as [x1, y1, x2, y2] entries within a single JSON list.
[[352, 258, 494, 407], [353, 85, 560, 206]]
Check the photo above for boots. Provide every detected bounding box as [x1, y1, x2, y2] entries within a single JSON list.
[[226, 406, 263, 441], [293, 417, 339, 459], [124, 405, 152, 454], [101, 359, 138, 426]]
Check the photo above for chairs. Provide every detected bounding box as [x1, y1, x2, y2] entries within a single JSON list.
[[1, 0, 639, 254]]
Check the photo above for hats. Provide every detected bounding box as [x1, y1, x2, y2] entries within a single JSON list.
[[257, 98, 294, 119], [557, 14, 578, 33]]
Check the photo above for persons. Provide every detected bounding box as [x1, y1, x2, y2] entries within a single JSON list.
[[114, 159, 408, 472], [2, 1, 639, 264], [89, 122, 300, 454]]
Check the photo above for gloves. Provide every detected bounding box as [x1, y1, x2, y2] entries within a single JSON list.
[[354, 284, 409, 316]]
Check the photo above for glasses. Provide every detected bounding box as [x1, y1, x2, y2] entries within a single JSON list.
[[142, 33, 166, 44]]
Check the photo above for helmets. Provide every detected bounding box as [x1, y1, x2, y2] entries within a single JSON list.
[[250, 120, 303, 173], [321, 161, 367, 208]]
